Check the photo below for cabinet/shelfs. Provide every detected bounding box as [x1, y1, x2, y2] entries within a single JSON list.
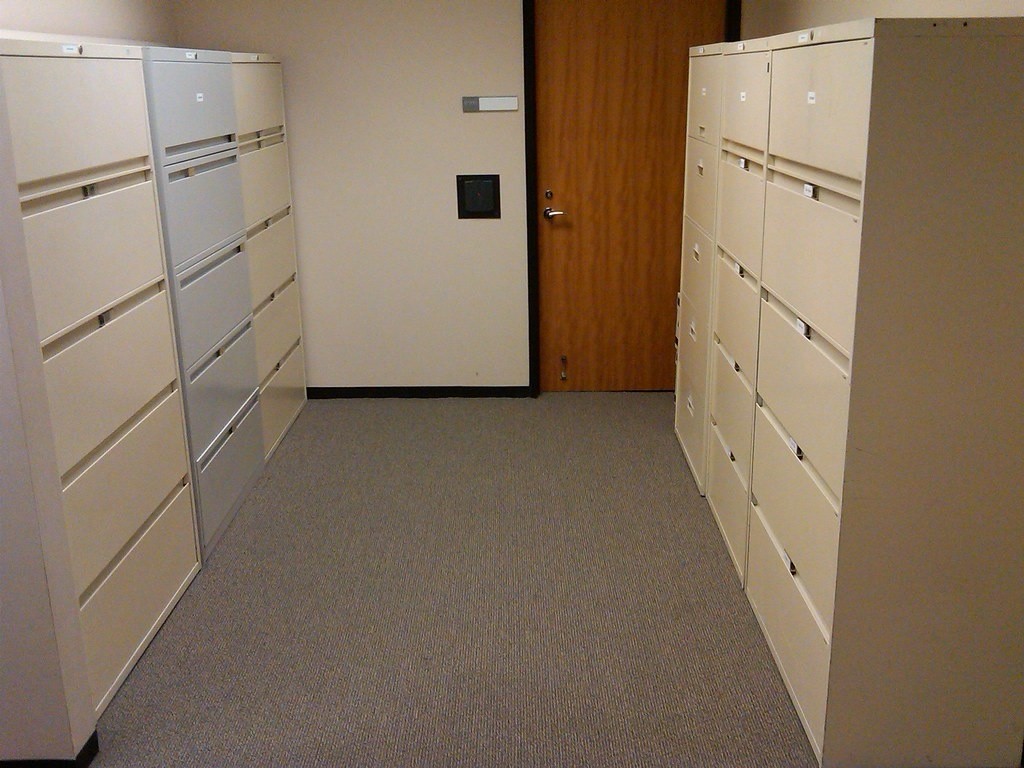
[[99, 36, 266, 564], [704, 36, 772, 589], [229, 51, 308, 462], [674, 43, 729, 499], [744, 17, 1024, 768], [0, 28, 203, 768]]
